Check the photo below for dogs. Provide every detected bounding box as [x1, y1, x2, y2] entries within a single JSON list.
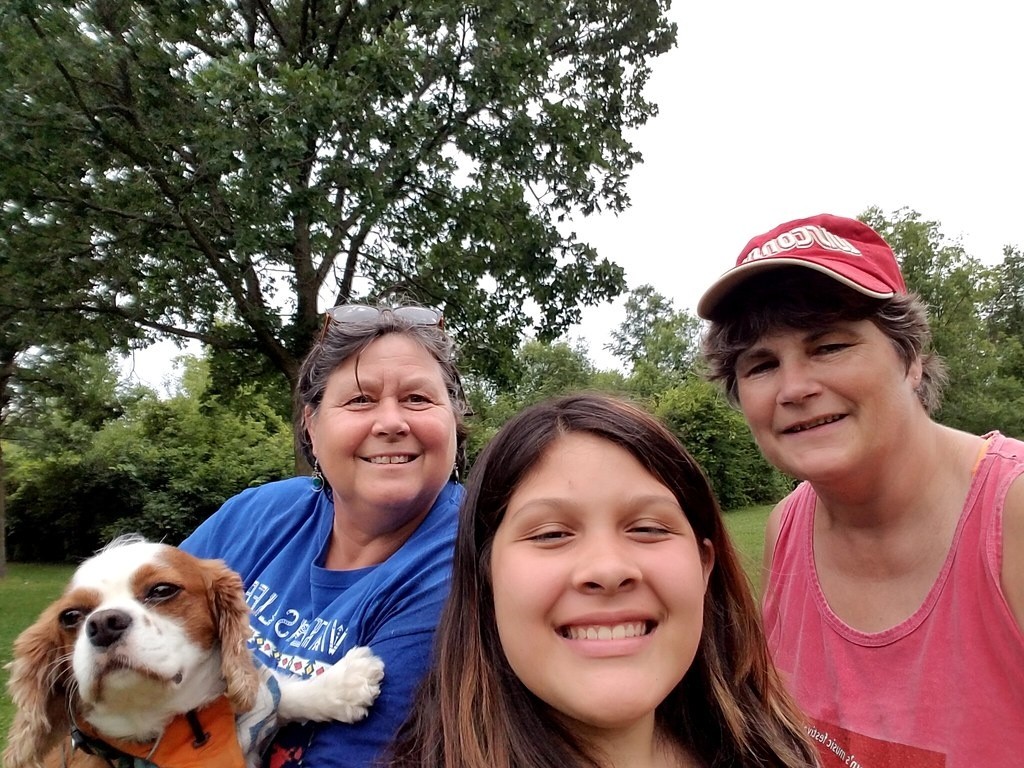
[[1, 532, 386, 768]]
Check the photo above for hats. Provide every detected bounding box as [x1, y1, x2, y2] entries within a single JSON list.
[[697, 213, 908, 321]]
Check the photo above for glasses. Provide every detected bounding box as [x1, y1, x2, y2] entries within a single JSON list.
[[319, 305, 444, 343]]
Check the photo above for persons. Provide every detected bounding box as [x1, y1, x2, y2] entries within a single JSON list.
[[693, 213, 1024, 768], [375, 399, 817, 768], [176, 304, 472, 768]]
